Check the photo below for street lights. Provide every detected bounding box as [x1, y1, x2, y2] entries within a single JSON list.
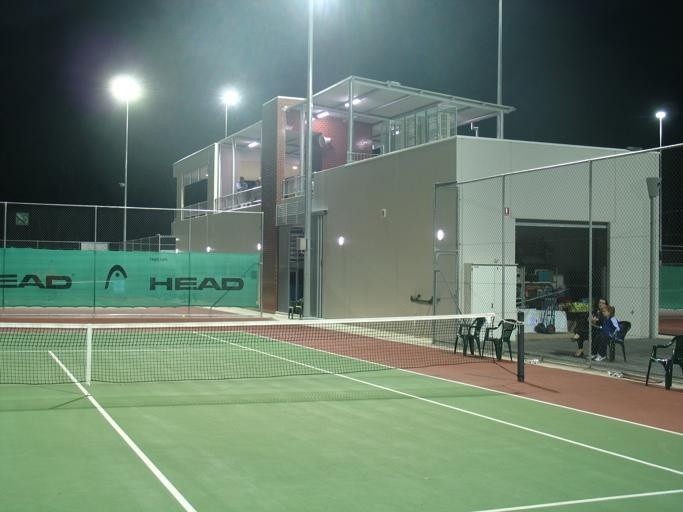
[[110, 74, 145, 249], [655, 109, 667, 263], [216, 85, 244, 138]]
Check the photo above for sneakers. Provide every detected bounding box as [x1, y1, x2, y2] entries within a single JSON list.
[[587, 352, 607, 362], [574, 350, 584, 357], [571, 337, 584, 344]]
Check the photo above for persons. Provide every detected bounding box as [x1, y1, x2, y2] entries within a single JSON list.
[[251, 179, 261, 204], [569, 297, 608, 357], [235, 176, 248, 192], [591, 305, 621, 362]]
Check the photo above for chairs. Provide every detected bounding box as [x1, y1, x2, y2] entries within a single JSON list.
[[482, 319, 516, 361], [454, 317, 485, 357], [609, 320, 631, 361], [645, 335, 683, 389]]
